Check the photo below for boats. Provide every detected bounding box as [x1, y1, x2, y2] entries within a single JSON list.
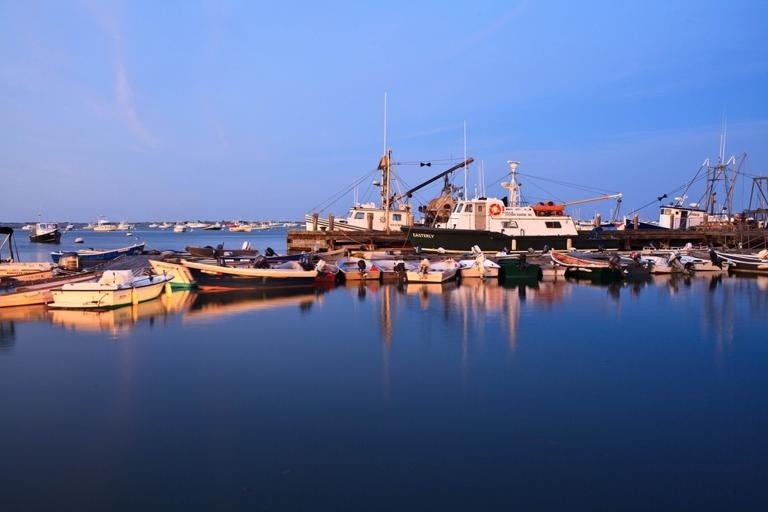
[[149, 221, 304, 232], [82, 217, 135, 231], [22, 222, 61, 242]]
